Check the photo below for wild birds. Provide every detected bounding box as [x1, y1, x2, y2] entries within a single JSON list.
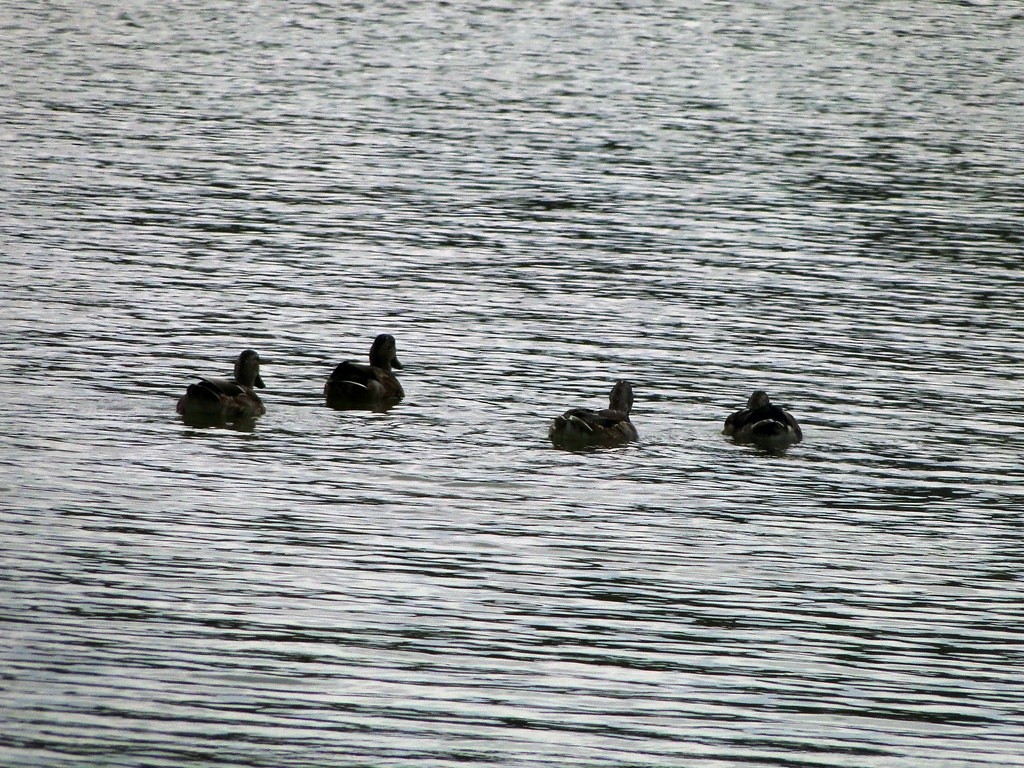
[[546, 377, 641, 451], [324, 331, 409, 414], [174, 347, 271, 425], [724, 388, 804, 445]]
